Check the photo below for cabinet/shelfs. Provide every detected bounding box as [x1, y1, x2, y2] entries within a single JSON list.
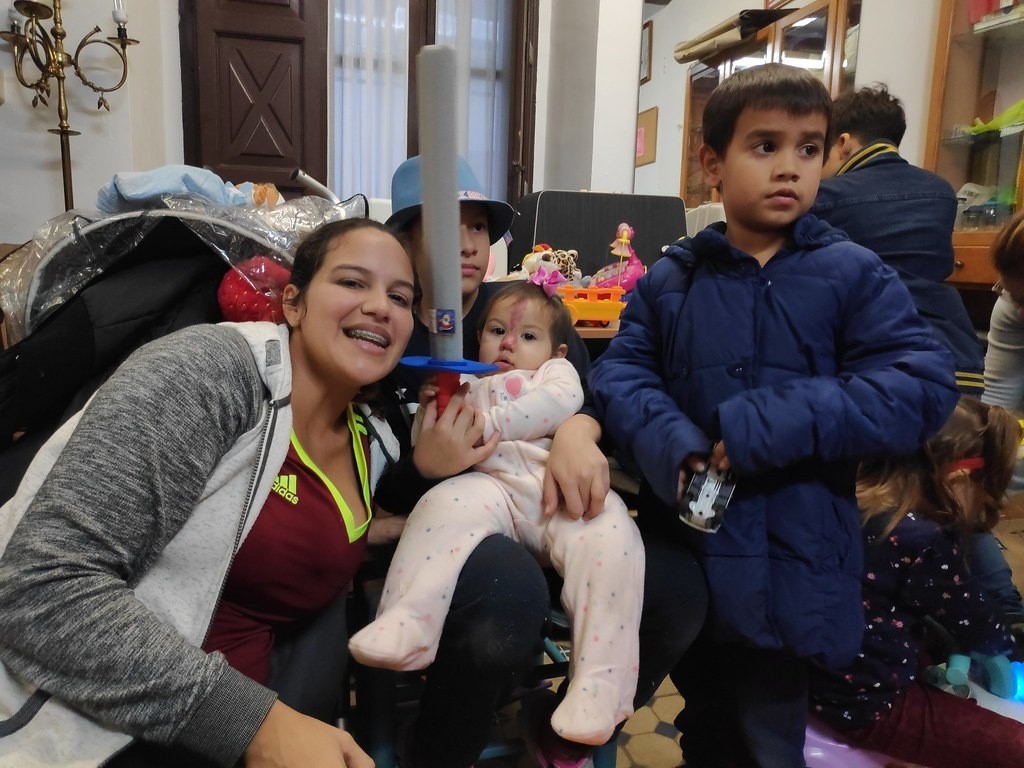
[[679, 1, 864, 215], [923, 0, 1023, 292]]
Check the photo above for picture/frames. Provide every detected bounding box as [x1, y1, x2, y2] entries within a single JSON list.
[[635, 105, 659, 168], [640, 19, 653, 86]]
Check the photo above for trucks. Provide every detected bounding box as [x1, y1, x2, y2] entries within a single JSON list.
[[553, 284, 629, 328]]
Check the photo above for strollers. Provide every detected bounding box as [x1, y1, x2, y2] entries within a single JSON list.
[[0, 170, 354, 498]]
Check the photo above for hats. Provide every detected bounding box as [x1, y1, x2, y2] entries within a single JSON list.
[[385, 152, 516, 248]]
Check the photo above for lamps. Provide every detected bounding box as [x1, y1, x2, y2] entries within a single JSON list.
[[0, 0, 140, 215]]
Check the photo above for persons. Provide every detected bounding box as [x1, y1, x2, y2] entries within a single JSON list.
[[0, 154, 707, 768], [586, 63, 1024, 768]]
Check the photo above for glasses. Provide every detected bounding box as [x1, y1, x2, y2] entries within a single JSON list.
[[991, 280, 1024, 312]]
